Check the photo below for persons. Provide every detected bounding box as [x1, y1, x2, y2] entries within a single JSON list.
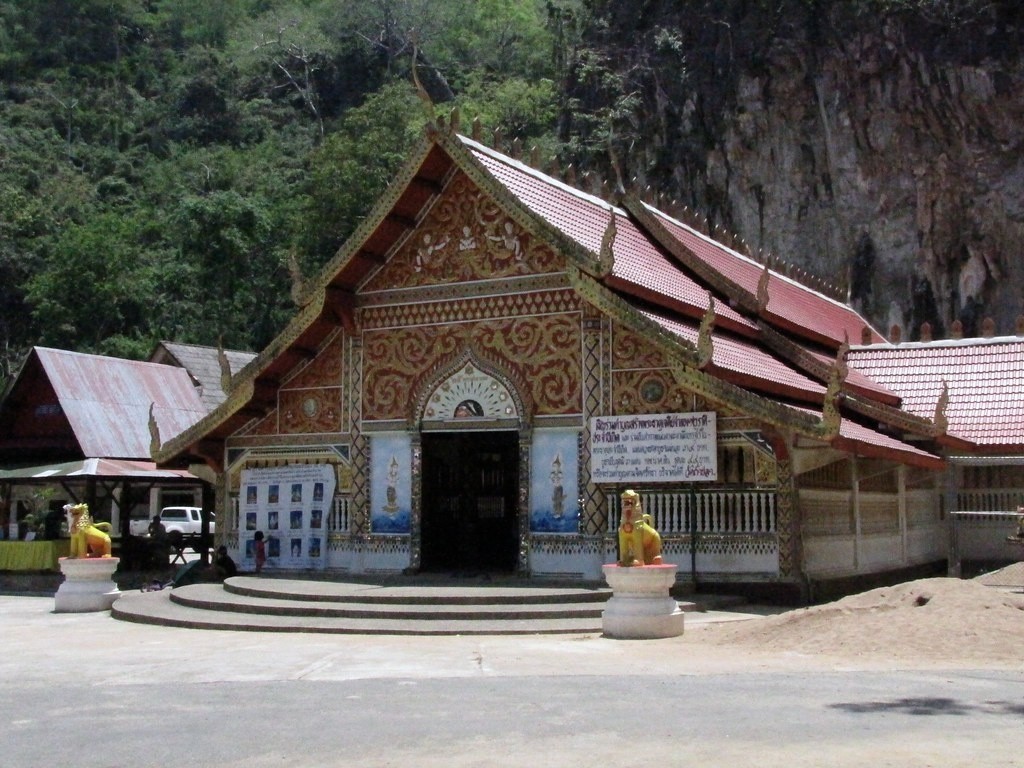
[[148, 515, 168, 553], [254, 531, 271, 576], [17, 500, 33, 541], [43, 507, 64, 541], [211, 545, 239, 582]]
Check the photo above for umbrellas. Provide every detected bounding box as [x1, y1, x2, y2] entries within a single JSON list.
[[174, 560, 202, 584]]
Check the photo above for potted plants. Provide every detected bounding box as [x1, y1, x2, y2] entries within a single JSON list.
[[15, 485, 62, 541]]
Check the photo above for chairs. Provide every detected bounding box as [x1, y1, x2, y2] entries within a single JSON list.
[[166, 531, 197, 566]]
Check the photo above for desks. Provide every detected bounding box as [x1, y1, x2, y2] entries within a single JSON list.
[[0, 539, 70, 574]]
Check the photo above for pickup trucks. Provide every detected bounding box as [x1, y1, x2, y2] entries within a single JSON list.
[[128, 506, 216, 544]]
